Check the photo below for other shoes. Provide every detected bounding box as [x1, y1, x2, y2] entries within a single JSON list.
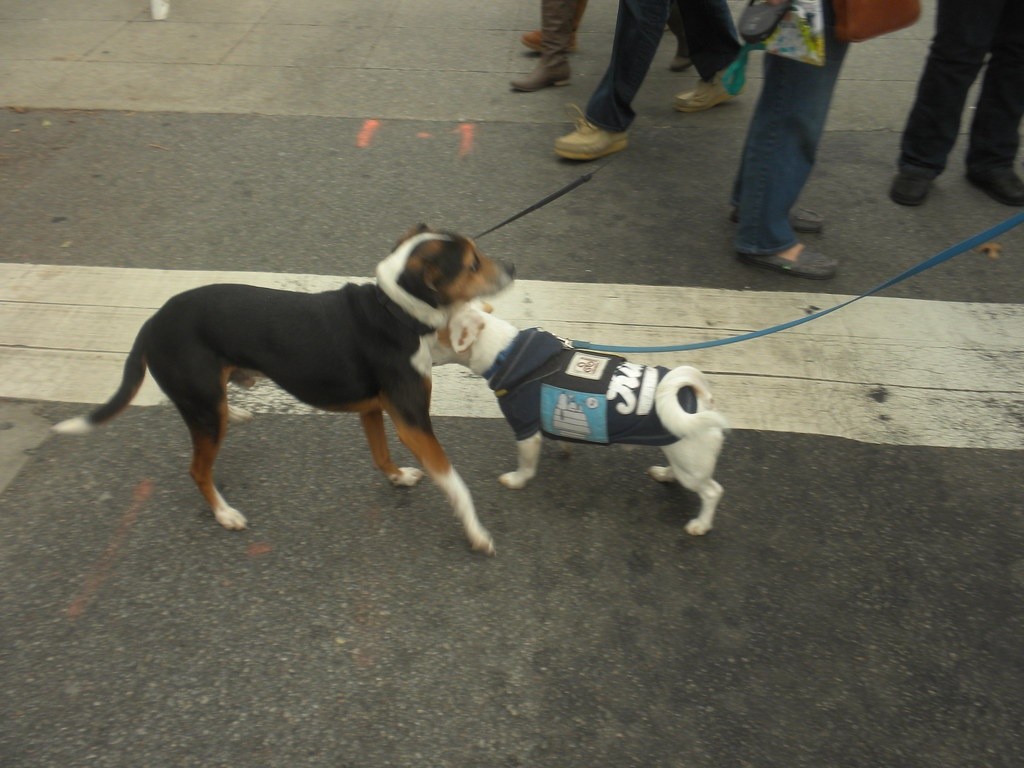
[[737, 236, 835, 280], [729, 201, 824, 234], [668, 39, 696, 71], [890, 167, 934, 206], [968, 161, 1024, 208], [520, 0, 588, 50]]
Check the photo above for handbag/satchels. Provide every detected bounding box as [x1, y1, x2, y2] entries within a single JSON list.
[[738, 0, 921, 66]]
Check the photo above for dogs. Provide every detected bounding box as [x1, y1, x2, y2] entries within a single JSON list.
[[427, 304, 728, 537], [51, 221, 515, 555]]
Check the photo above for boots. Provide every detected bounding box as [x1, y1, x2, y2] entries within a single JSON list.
[[510, 0, 590, 90]]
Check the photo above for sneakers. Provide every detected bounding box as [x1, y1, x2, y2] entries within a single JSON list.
[[671, 67, 747, 112], [553, 103, 627, 160]]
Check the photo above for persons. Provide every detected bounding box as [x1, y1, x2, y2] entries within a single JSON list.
[[890, 0, 1024, 207], [511, 0, 741, 159], [731, 0, 837, 278]]
